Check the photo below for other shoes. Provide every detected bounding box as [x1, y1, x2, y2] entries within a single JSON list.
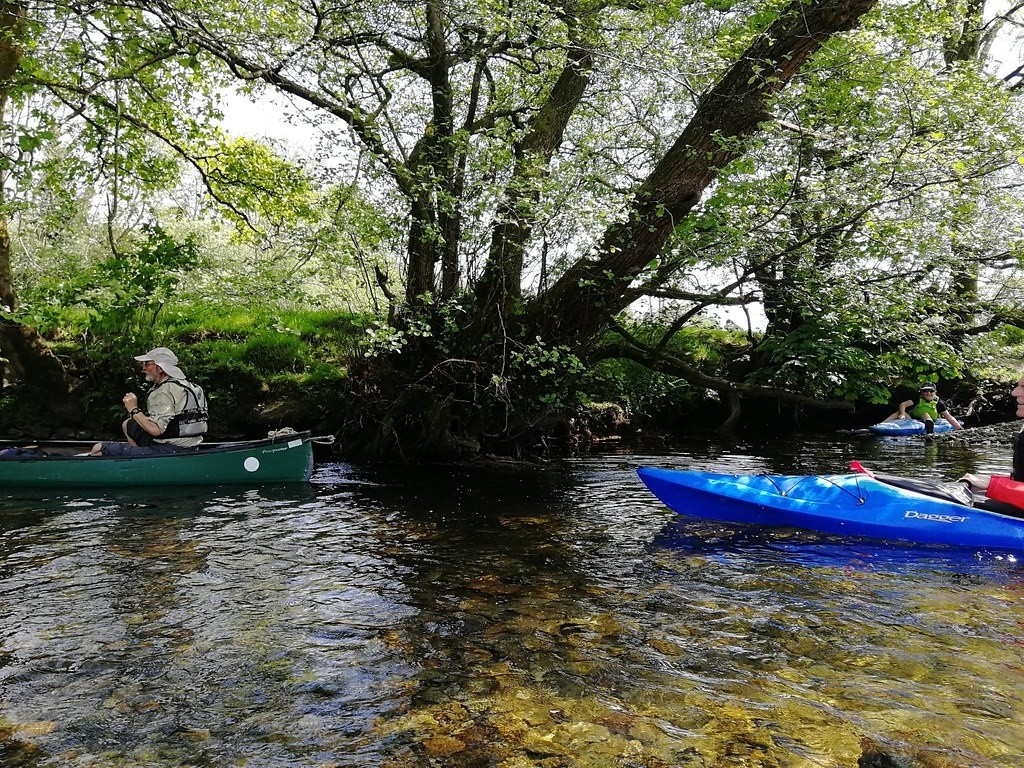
[[925, 437, 933, 443], [925, 419, 934, 435]]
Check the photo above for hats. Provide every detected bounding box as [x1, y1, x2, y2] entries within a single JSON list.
[[134, 347, 186, 380], [920, 382, 936, 391]]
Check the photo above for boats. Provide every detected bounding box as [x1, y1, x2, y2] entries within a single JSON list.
[[635, 463, 1024, 564], [981, 473, 1024, 512], [0, 419, 314, 493], [867, 416, 965, 437]]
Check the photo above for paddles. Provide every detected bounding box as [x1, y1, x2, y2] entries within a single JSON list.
[[928, 479, 975, 508]]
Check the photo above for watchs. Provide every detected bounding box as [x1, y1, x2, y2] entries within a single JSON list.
[[130, 407, 143, 418]]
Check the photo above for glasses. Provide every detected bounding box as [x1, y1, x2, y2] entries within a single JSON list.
[[142, 361, 156, 368], [922, 389, 935, 393]]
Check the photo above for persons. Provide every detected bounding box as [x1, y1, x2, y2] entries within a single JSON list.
[[881, 382, 965, 443], [89, 347, 204, 456], [958, 375, 1024, 510]]
